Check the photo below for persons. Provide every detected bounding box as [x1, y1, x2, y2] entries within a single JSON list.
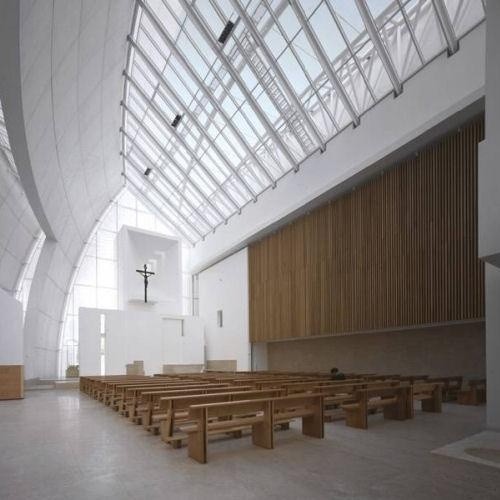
[[331, 368, 344, 380]]
